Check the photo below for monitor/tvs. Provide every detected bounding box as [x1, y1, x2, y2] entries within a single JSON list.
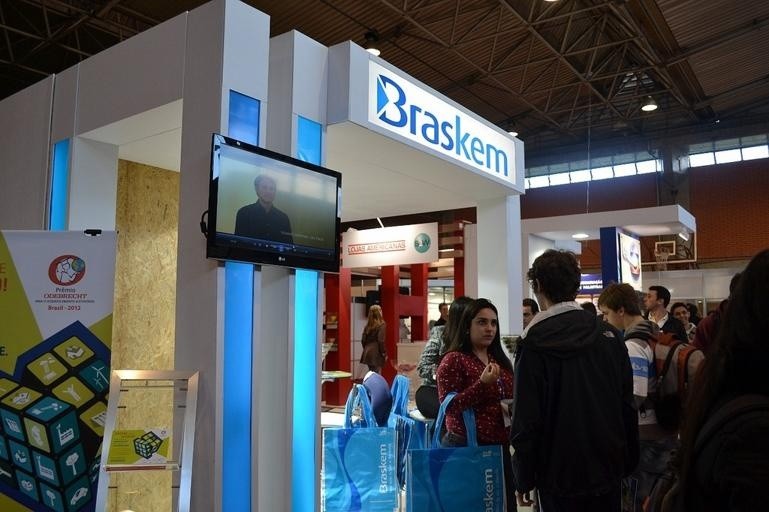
[[205, 133, 342, 273]]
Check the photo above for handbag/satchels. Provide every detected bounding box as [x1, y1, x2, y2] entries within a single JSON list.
[[322, 384, 397, 512], [404, 391, 507, 512], [353, 375, 426, 491]]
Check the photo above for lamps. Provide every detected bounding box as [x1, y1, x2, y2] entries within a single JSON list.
[[363, 32, 381, 55], [641, 95, 659, 112], [503, 120, 519, 137]]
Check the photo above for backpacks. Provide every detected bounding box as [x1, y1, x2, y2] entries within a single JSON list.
[[630, 329, 707, 439]]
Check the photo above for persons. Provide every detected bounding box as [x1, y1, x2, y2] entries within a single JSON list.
[[235, 175, 293, 243], [417, 250, 769, 511], [360, 304, 388, 376]]
